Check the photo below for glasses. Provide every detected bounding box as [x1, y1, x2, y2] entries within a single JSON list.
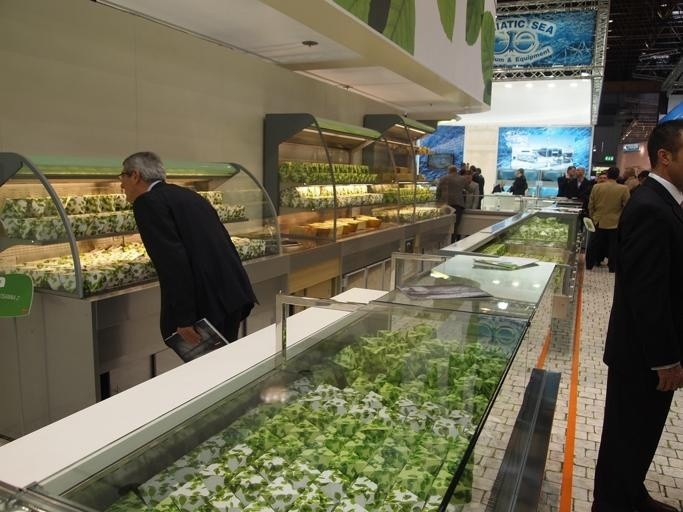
[[117, 172, 129, 180]]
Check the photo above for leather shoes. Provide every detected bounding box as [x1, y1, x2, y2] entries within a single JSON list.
[[641, 496, 679, 512]]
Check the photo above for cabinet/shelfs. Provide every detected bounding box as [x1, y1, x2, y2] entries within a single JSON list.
[[260, 110, 466, 284], [0, 147, 281, 441]]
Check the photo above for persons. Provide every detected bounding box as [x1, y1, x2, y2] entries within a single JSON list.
[[590, 119, 683, 512], [556, 165, 649, 272], [492, 180, 505, 193], [436, 165, 475, 242], [507, 169, 527, 196], [458, 162, 484, 209], [119, 151, 259, 360]]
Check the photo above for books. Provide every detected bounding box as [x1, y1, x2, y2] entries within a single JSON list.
[[163, 317, 230, 362]]
